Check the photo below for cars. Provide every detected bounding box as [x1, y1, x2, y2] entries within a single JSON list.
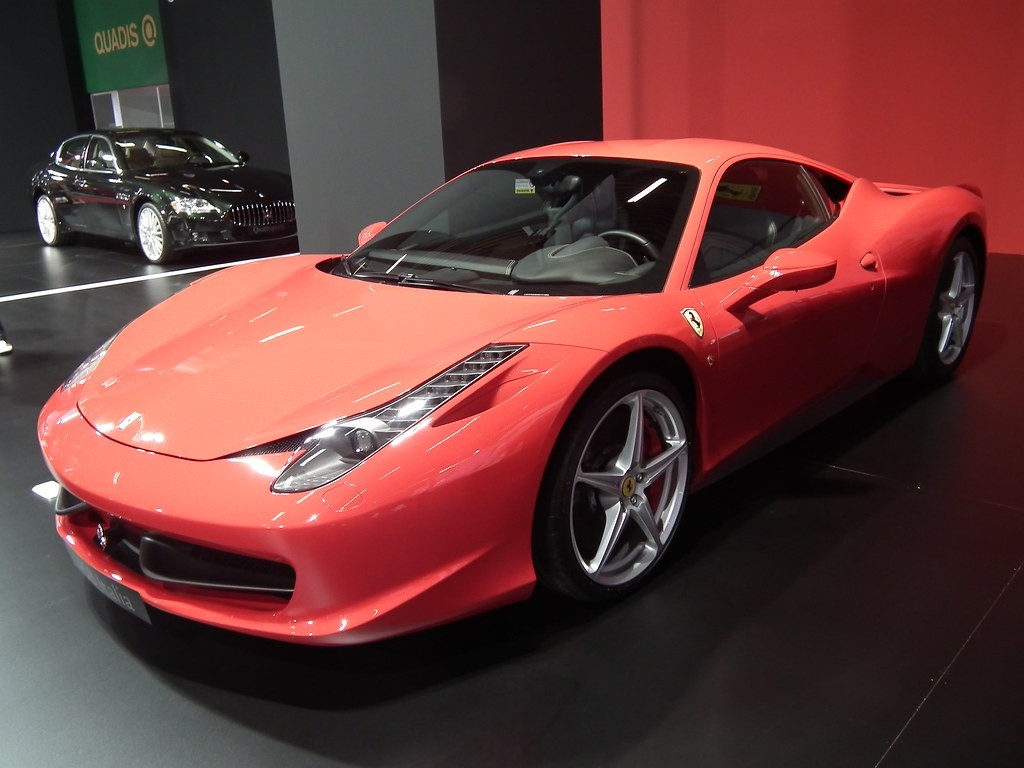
[[29, 125, 299, 266]]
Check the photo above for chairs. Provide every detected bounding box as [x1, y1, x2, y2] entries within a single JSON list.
[[543, 167, 620, 253], [691, 185, 806, 289]]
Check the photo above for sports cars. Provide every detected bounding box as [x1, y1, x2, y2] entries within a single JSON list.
[[37, 138, 989, 649]]
